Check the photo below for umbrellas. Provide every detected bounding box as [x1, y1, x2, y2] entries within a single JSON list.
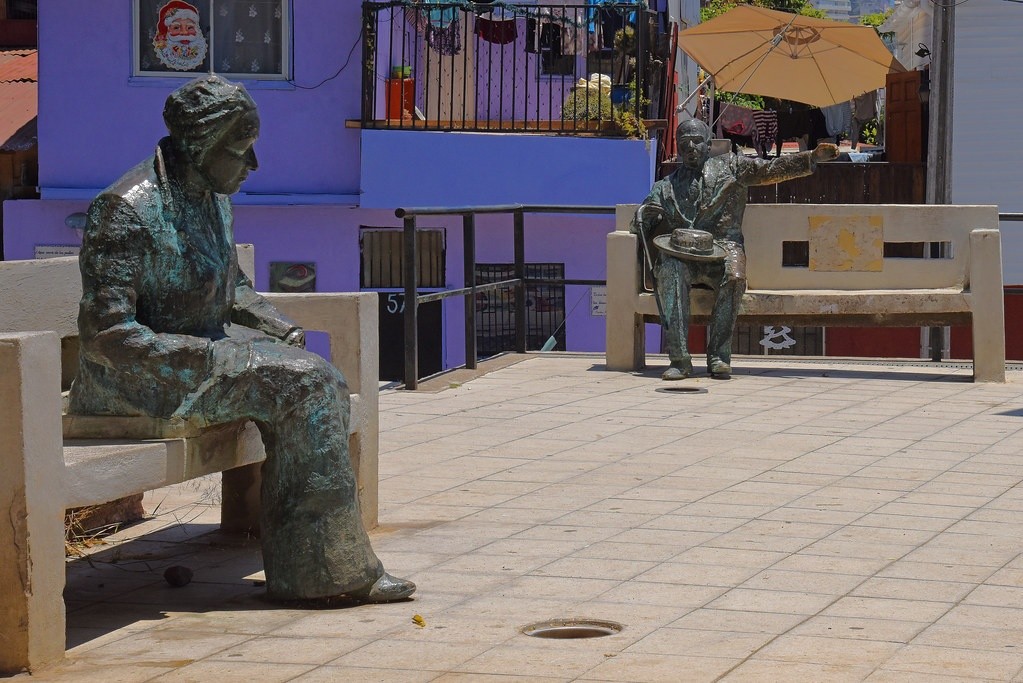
[[673, 3, 907, 115]]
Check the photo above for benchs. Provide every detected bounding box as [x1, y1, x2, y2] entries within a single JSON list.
[[0, 242, 381, 673], [605, 204, 1006, 384]]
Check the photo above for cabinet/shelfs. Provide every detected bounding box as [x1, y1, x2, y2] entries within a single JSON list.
[[885, 71, 929, 163], [385, 78, 414, 119]]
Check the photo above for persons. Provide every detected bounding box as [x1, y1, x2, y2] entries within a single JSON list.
[[630, 118, 840, 380], [67, 72, 416, 609]]
